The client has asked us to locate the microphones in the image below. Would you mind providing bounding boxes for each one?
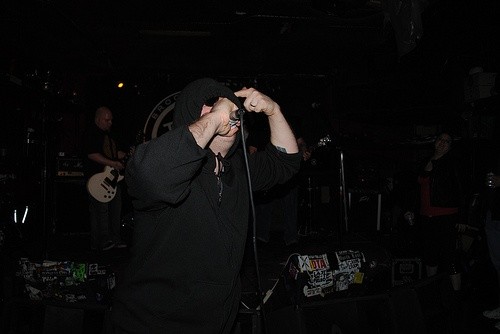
[230,109,245,121]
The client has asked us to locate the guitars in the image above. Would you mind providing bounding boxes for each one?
[301,134,330,159]
[87,136,150,203]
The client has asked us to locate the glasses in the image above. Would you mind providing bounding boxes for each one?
[205,96,219,106]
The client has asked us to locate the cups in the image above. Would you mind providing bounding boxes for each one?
[486,173,495,191]
[449,273,462,290]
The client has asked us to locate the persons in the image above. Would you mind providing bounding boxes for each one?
[111,78,304,334]
[254,79,311,251]
[83,106,135,250]
[398,134,500,329]
